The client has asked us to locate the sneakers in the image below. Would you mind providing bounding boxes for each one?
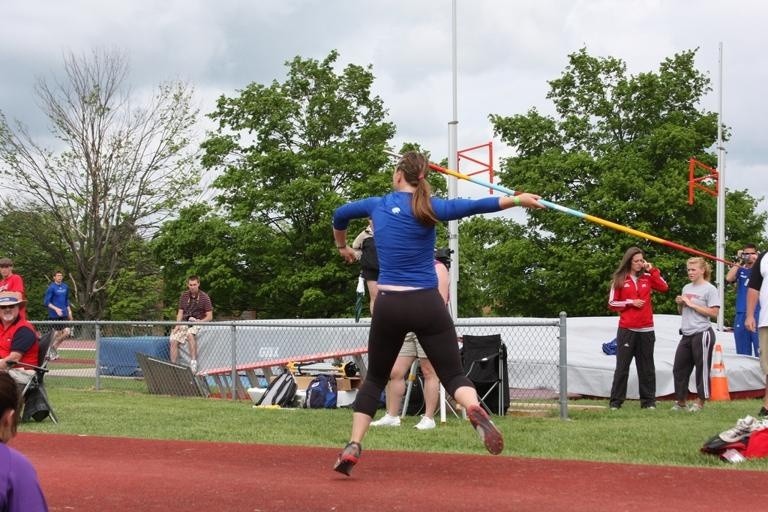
[190,360,198,374]
[688,403,704,412]
[465,404,505,456]
[758,407,768,418]
[719,415,761,443]
[413,417,436,430]
[47,348,60,361]
[369,412,401,427]
[332,441,361,476]
[670,403,688,412]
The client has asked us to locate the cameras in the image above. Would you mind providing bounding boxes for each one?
[69,324,74,337]
[742,253,749,260]
[186,315,195,327]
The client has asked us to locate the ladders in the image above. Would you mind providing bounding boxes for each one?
[194,347,368,401]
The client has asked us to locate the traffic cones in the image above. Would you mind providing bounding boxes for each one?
[708,344,733,401]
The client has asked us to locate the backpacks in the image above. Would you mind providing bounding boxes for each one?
[303,375,338,409]
[255,372,297,408]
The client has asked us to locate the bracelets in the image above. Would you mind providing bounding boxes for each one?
[513,193,521,208]
[335,242,348,251]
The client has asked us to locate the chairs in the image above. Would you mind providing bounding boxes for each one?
[0,326,61,427]
[385,331,510,421]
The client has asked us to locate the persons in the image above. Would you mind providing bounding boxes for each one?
[166,275,214,375]
[0,255,29,322]
[604,246,671,413]
[0,289,41,391]
[42,270,74,362]
[353,212,376,317]
[741,247,768,418]
[670,254,722,413]
[723,243,761,358]
[324,148,549,479]
[368,251,449,431]
[0,370,54,512]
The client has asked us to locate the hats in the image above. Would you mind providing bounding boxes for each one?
[0,291,28,307]
